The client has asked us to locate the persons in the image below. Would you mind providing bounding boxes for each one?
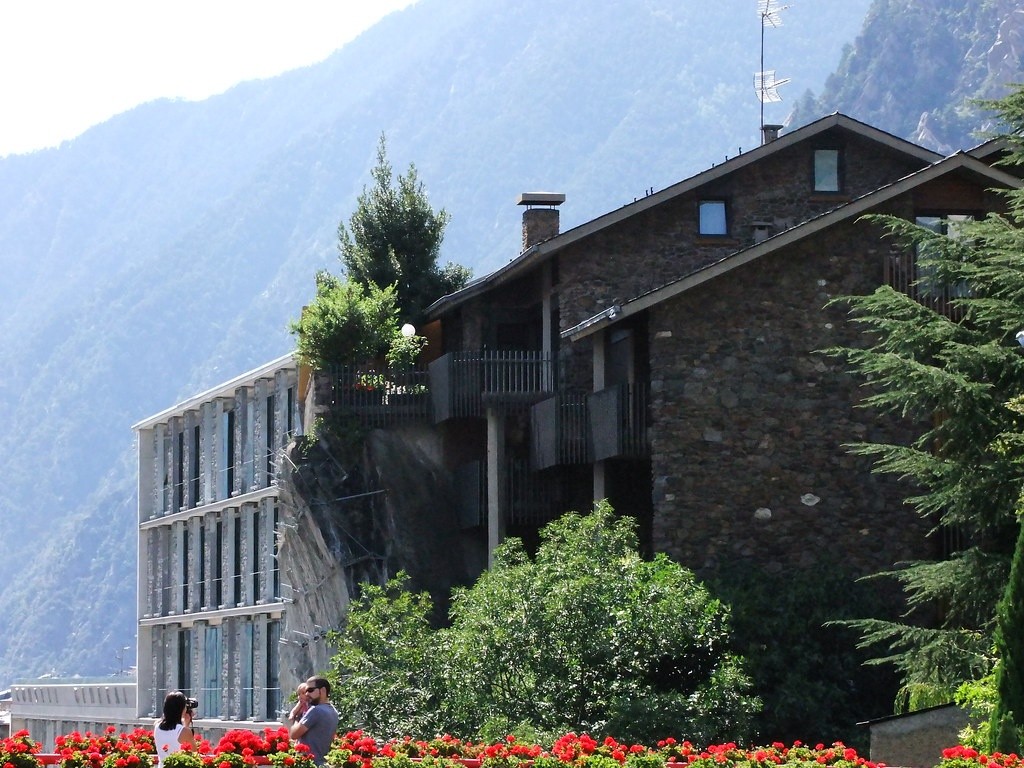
[288,676,339,768]
[154,691,197,768]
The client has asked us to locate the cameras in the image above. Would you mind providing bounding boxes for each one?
[186,697,199,714]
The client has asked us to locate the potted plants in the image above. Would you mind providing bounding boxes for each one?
[388,382,430,404]
[354,373,386,405]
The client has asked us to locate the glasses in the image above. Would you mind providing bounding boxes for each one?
[306,687,319,693]
